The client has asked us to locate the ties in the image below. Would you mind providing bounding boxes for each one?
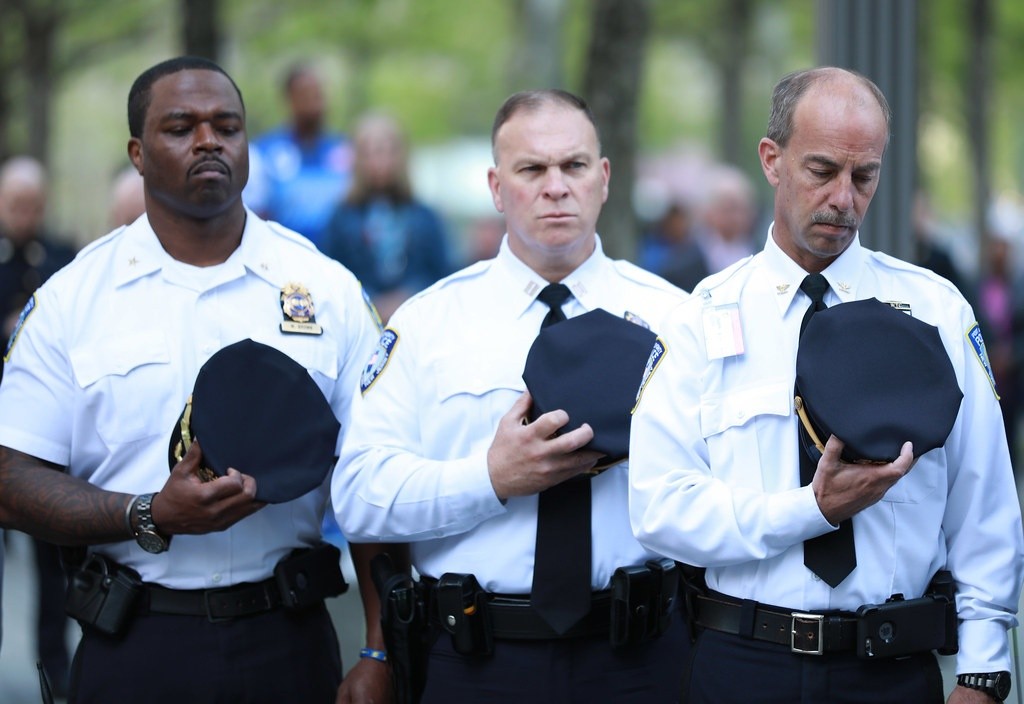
[531,283,595,634]
[799,273,857,589]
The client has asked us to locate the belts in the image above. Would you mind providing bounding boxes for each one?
[483,599,625,641]
[137,583,283,617]
[691,594,856,652]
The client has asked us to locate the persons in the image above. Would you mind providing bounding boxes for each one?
[247,64,506,324]
[629,67,1024,704]
[0,159,145,357]
[331,90,689,703]
[639,174,757,292]
[0,58,392,704]
[917,184,1024,458]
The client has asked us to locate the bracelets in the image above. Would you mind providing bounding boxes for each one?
[125,495,139,535]
[359,648,389,663]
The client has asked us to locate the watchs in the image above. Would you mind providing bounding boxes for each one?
[135,492,172,554]
[958,671,1011,701]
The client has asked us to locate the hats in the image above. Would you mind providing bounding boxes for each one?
[793,296,964,466]
[522,307,663,478]
[169,337,342,518]
